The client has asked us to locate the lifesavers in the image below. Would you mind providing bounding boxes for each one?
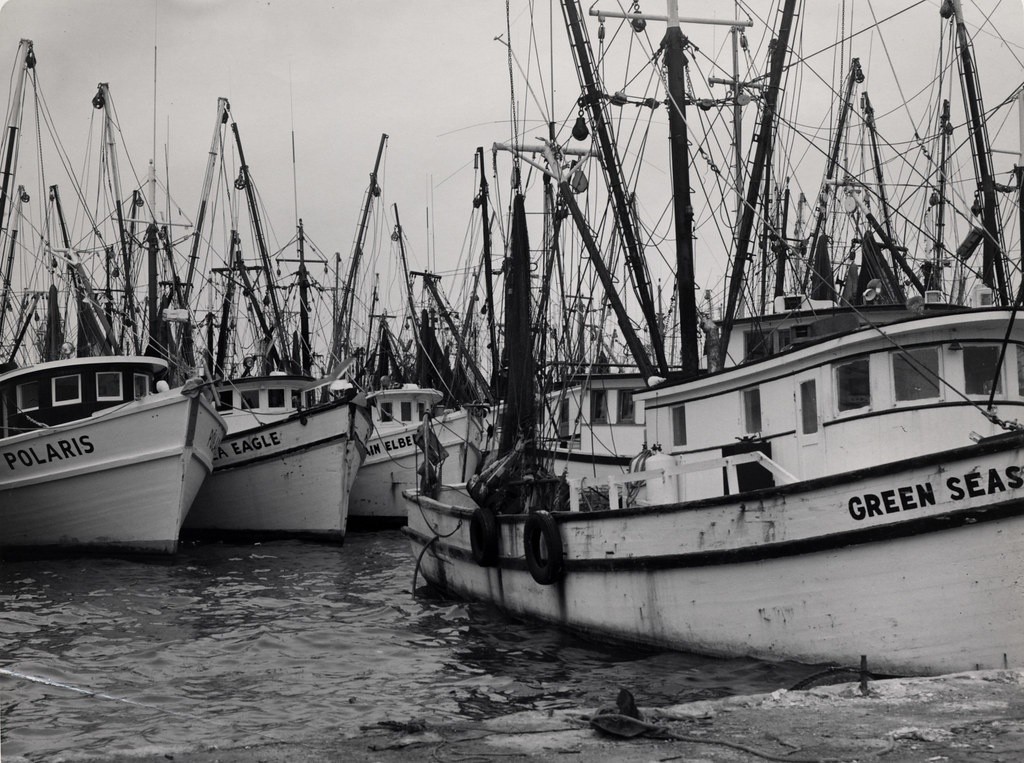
[523,508,564,585]
[468,506,500,568]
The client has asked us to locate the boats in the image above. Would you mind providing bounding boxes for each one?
[0,2,1023,676]
